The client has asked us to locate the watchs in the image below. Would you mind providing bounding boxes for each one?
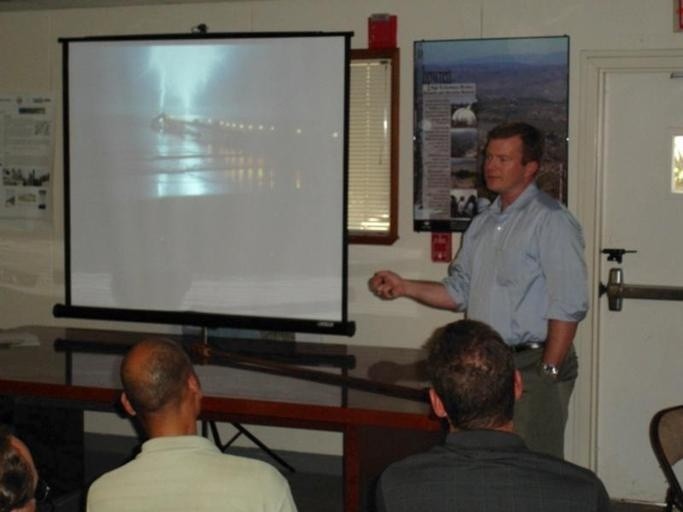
[538,359,561,377]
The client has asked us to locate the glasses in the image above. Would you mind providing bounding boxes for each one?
[29,480,50,504]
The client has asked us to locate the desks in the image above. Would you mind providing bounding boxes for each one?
[0,325,438,511]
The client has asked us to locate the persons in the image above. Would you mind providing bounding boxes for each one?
[366,122,590,461]
[373,319,612,511]
[0,428,44,512]
[84,339,298,512]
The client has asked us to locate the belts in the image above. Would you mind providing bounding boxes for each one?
[511,341,544,354]
